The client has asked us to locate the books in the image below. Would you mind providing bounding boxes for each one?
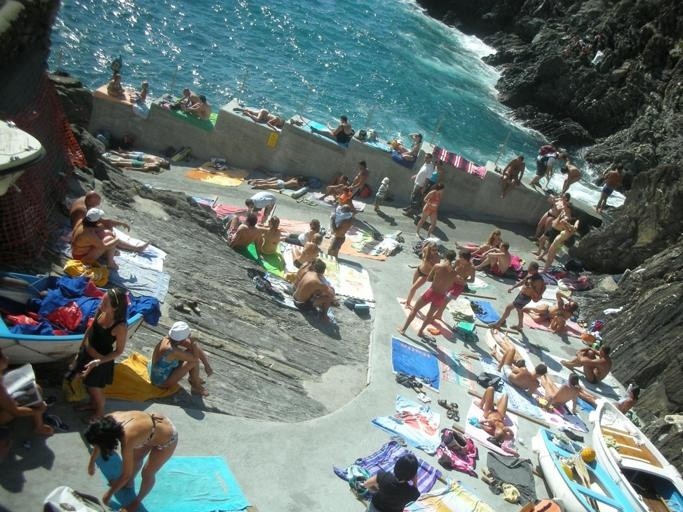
[2,362,43,408]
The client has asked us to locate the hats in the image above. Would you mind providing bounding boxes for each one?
[86,208,104,222]
[169,321,191,342]
[381,177,390,186]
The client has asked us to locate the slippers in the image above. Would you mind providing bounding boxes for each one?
[578,320,583,327]
[44,396,58,406]
[450,402,459,409]
[582,320,588,328]
[44,413,70,433]
[431,337,437,347]
[185,299,201,314]
[412,380,426,393]
[438,399,452,409]
[172,303,191,312]
[421,335,431,343]
[411,381,420,392]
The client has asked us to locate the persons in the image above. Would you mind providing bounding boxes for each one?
[491,344,549,398]
[593,161,624,212]
[149,321,213,395]
[77,288,128,422]
[454,229,511,276]
[375,177,390,211]
[292,258,338,323]
[501,156,526,196]
[490,327,526,368]
[401,153,442,218]
[245,192,276,225]
[577,381,640,414]
[103,72,211,172]
[399,239,440,309]
[281,218,320,246]
[432,251,475,320]
[396,250,459,337]
[292,233,323,268]
[364,454,421,512]
[562,24,606,59]
[249,176,305,190]
[257,216,281,256]
[474,386,514,442]
[309,118,352,144]
[71,190,150,253]
[561,345,613,383]
[541,372,582,414]
[327,205,352,257]
[319,176,349,207]
[83,410,178,512]
[70,208,123,270]
[344,161,368,214]
[485,260,543,330]
[417,183,444,237]
[0,348,55,436]
[12,387,35,406]
[231,105,285,132]
[393,132,422,162]
[530,149,581,195]
[224,213,262,261]
[523,291,578,330]
[529,193,581,272]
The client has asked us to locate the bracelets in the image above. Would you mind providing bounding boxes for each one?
[94,359,99,367]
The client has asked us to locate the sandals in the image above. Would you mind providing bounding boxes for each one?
[447,409,453,420]
[453,410,458,421]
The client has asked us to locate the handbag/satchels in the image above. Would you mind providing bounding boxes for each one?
[441,428,468,455]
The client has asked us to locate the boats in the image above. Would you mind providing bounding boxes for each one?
[534,428,632,512]
[0,271,159,364]
[591,396,682,512]
[0,117,48,197]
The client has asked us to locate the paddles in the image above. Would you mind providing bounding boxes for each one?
[537,397,570,421]
[572,456,598,512]
[578,456,600,512]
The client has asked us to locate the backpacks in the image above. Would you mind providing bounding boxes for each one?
[539,144,557,156]
